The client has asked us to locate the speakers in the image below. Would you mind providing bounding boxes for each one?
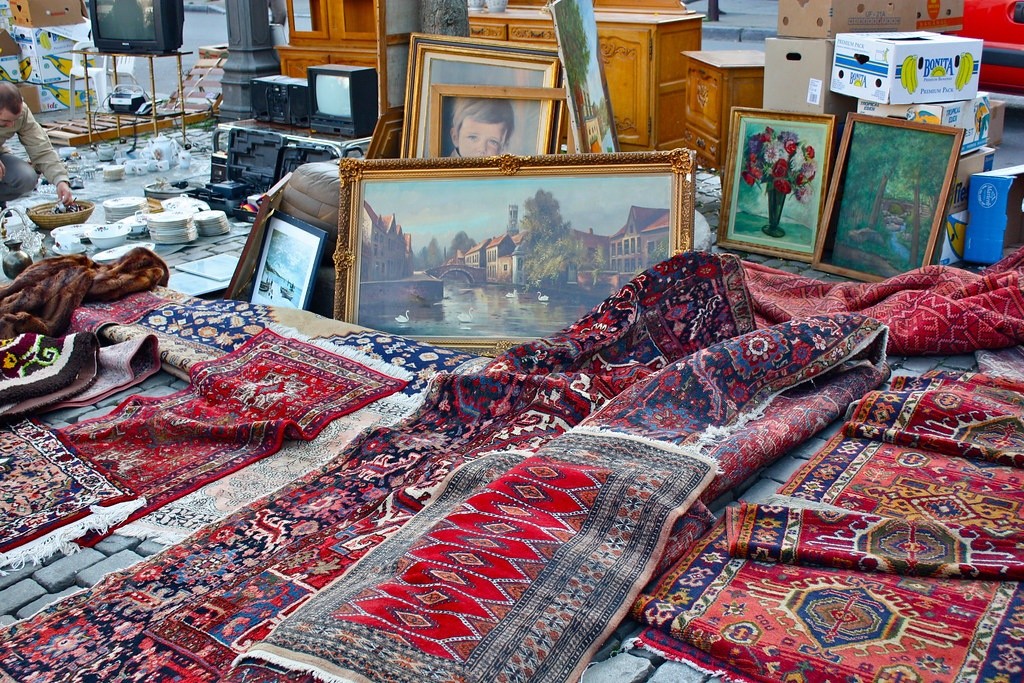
[250,75,290,121]
[286,80,311,128]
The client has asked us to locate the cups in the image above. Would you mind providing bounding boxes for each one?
[117,157,170,175]
[69,162,96,179]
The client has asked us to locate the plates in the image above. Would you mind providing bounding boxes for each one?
[52,244,87,256]
[93,242,156,264]
[103,180,231,244]
[50,224,97,241]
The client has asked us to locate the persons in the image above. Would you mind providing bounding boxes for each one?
[0,81,74,217]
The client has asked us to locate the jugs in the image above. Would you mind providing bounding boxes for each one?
[147,133,178,164]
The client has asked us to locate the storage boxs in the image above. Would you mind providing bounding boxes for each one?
[761,0,1024,265]
[198,43,229,60]
[0,0,95,113]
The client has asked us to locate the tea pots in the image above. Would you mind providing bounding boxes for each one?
[90,144,117,162]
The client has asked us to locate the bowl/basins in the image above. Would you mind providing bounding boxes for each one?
[89,225,131,249]
[26,201,95,229]
[57,236,82,250]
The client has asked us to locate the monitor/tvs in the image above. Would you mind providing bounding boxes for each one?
[90,0,184,54]
[306,64,379,139]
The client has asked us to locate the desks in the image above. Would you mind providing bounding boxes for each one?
[69,50,194,146]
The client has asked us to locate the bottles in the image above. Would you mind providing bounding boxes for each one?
[2,240,33,280]
[179,148,191,169]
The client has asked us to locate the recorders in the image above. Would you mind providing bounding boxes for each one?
[108,84,153,115]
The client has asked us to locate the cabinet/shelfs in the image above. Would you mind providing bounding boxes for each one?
[467,0,706,152]
[680,50,764,170]
[273,0,378,119]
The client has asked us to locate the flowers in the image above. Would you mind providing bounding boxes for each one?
[740,124,817,203]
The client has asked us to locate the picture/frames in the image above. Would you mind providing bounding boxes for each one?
[717,103,836,263]
[223,172,330,310]
[335,32,697,356]
[813,112,966,284]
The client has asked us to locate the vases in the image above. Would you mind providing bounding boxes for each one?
[761,181,787,238]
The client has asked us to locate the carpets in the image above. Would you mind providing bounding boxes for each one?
[1,251,1024,683]
